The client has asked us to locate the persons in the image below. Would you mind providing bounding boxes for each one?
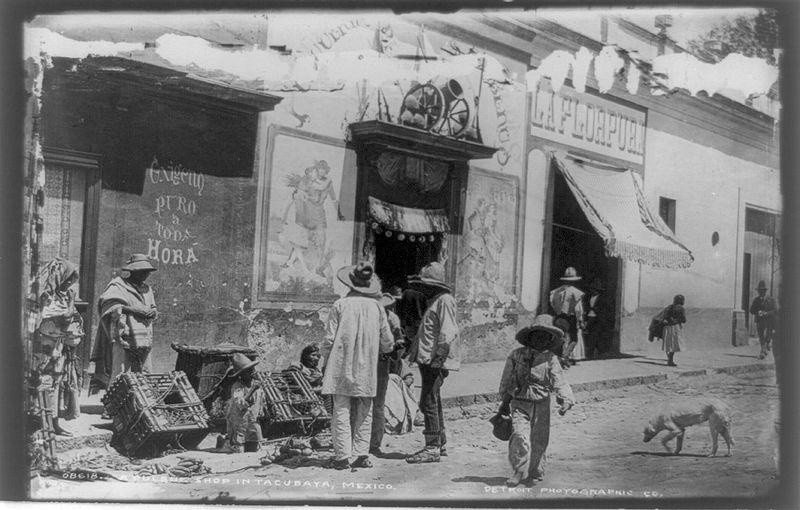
[552,266,618,362]
[497,323,575,486]
[278,160,338,273]
[25,254,457,471]
[749,277,783,362]
[657,293,688,369]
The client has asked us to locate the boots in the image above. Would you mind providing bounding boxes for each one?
[406,430,441,463]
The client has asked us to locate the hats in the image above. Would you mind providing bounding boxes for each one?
[755,280,767,290]
[120,253,158,274]
[560,267,582,282]
[338,262,381,295]
[515,314,564,348]
[406,261,453,294]
[229,350,259,378]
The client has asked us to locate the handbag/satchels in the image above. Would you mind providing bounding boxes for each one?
[489,415,513,441]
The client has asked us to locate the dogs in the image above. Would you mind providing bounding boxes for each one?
[640,397,737,458]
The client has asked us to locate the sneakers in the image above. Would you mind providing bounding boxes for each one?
[507,471,523,486]
